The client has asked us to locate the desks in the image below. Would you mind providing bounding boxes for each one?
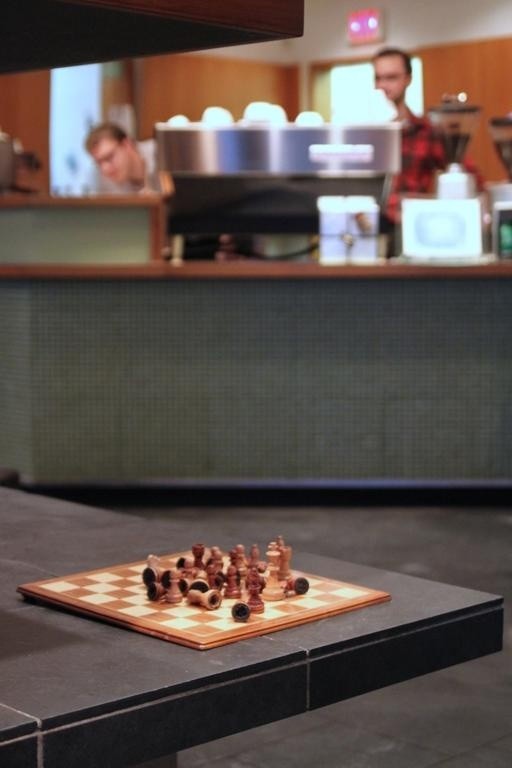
[1,484,503,768]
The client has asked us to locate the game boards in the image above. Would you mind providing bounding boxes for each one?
[16,545,393,654]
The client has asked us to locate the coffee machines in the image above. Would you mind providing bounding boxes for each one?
[425,91,511,204]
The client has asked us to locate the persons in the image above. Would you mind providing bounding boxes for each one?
[372,48,485,258]
[79,123,158,194]
